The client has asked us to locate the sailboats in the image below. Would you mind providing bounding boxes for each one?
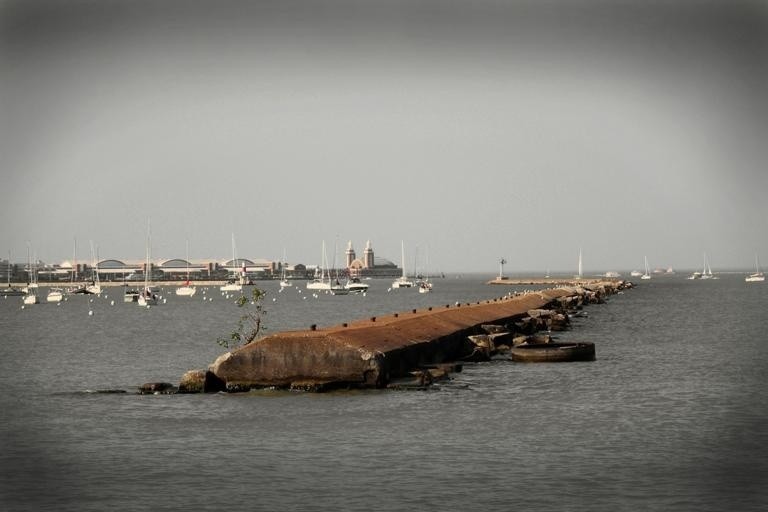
[306,241,331,290]
[327,246,349,296]
[175,239,196,297]
[65,239,104,295]
[21,246,39,306]
[3,243,26,297]
[122,232,162,307]
[279,249,294,288]
[219,234,243,292]
[495,249,765,286]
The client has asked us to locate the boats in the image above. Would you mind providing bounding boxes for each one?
[224,274,235,283]
[46,291,64,303]
[345,276,370,294]
[391,240,416,290]
[414,273,433,294]
[239,276,254,286]
